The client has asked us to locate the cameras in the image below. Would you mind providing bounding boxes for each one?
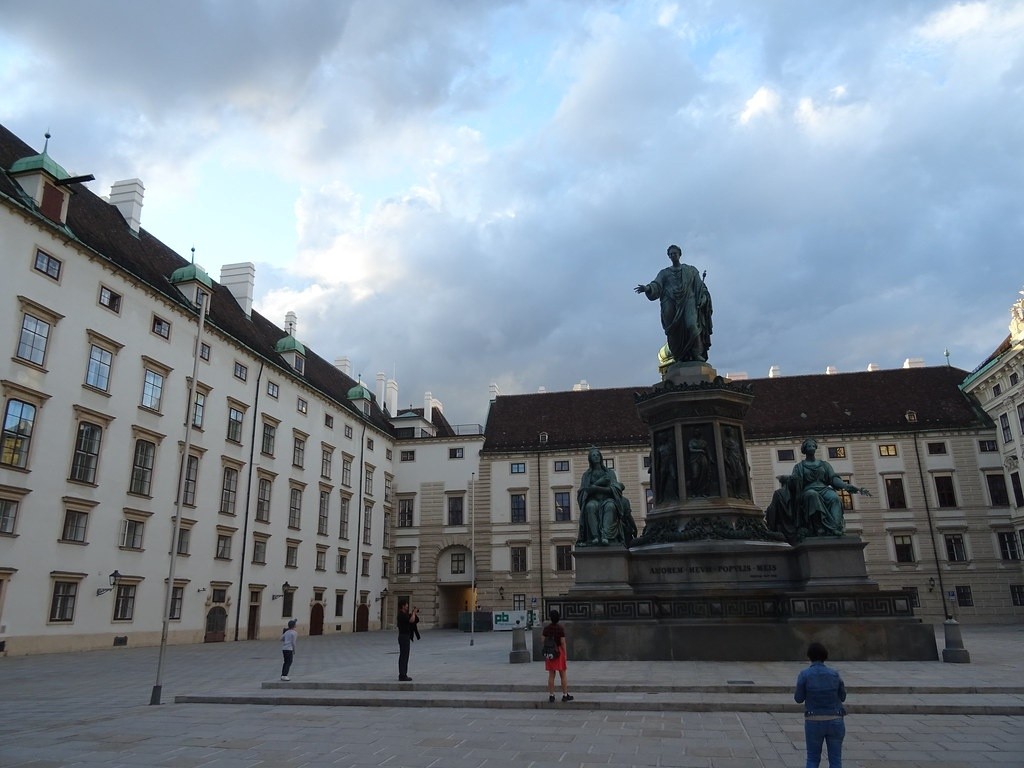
[414,607,419,612]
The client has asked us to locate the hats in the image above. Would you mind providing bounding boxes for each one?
[288,619,297,628]
[549,610,560,623]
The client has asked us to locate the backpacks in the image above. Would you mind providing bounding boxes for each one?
[542,624,560,659]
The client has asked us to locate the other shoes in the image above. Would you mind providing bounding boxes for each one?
[280,675,290,680]
[399,674,411,680]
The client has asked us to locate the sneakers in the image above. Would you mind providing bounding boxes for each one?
[549,695,555,701]
[562,693,574,702]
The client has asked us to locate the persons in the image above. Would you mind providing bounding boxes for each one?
[577,448,626,547]
[280,619,298,681]
[541,609,574,703]
[634,244,709,363]
[775,436,858,537]
[397,599,420,682]
[794,641,846,768]
